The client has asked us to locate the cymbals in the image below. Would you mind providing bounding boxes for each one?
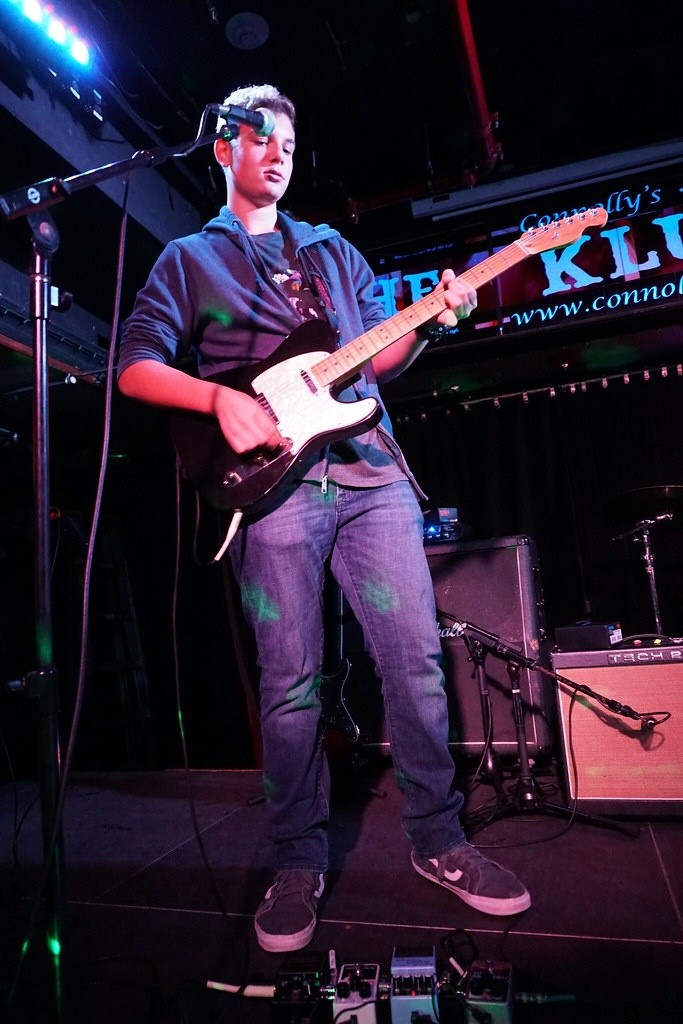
[598,483,683,532]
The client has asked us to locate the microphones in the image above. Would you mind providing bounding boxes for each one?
[209,103,276,138]
[641,715,657,731]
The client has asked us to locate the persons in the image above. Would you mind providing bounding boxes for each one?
[116,85,531,954]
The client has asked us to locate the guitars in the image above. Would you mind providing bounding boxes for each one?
[166,201,613,524]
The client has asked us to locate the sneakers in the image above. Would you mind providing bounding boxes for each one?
[410,840,533,917]
[254,863,326,951]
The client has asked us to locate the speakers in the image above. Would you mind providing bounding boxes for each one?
[548,644,683,818]
[334,532,557,764]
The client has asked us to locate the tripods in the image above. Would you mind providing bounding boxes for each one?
[431,609,643,842]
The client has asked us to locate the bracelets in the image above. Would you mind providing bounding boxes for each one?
[209,384,223,414]
